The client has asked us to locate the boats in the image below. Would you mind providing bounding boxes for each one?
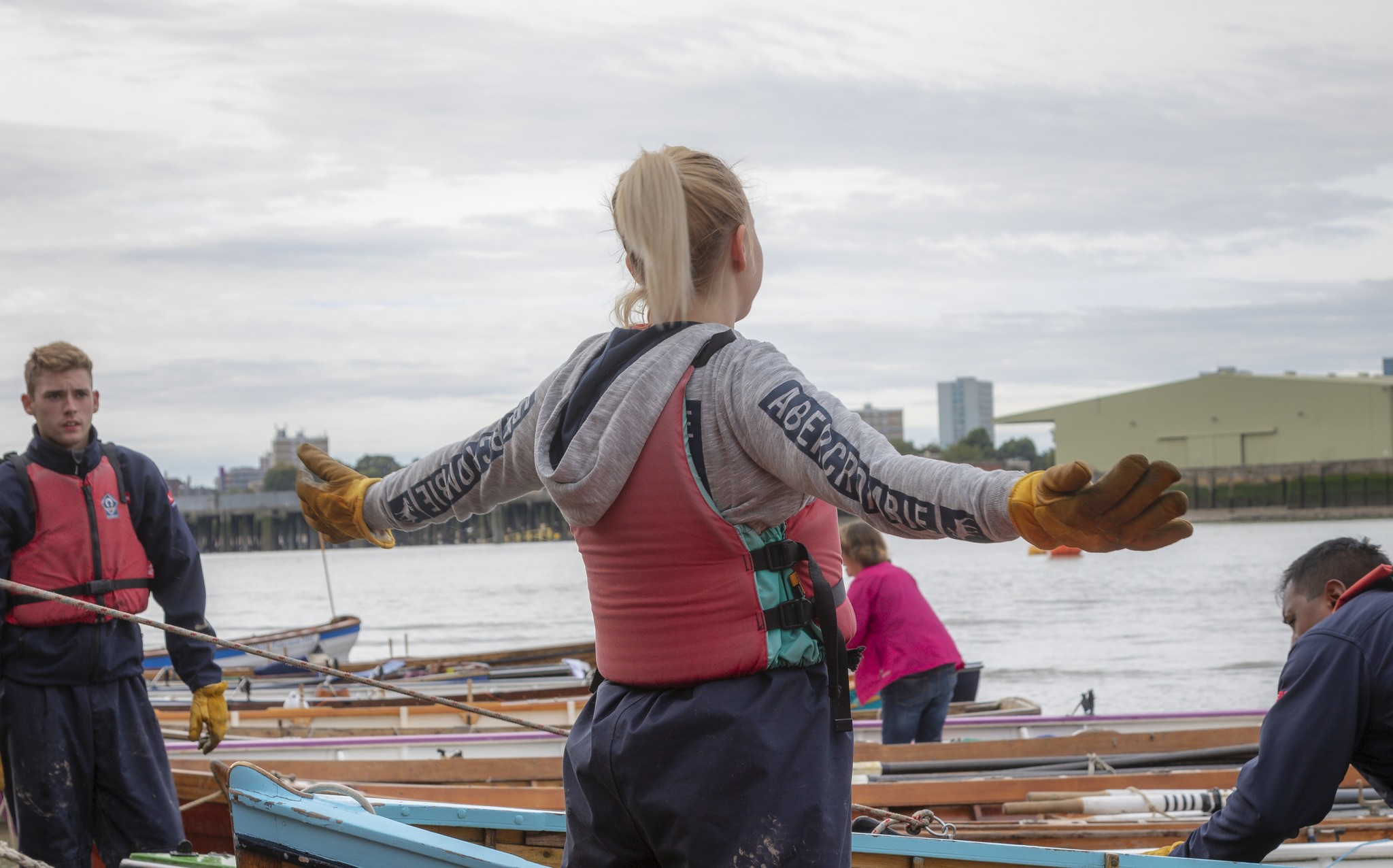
[137,608,1393,866]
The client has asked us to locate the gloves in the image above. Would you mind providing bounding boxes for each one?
[1140,839,1185,857]
[296,443,396,550]
[188,681,230,756]
[1006,453,1193,553]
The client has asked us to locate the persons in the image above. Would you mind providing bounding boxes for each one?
[0,339,229,868]
[835,520,966,746]
[297,148,1195,868]
[1138,535,1393,863]
